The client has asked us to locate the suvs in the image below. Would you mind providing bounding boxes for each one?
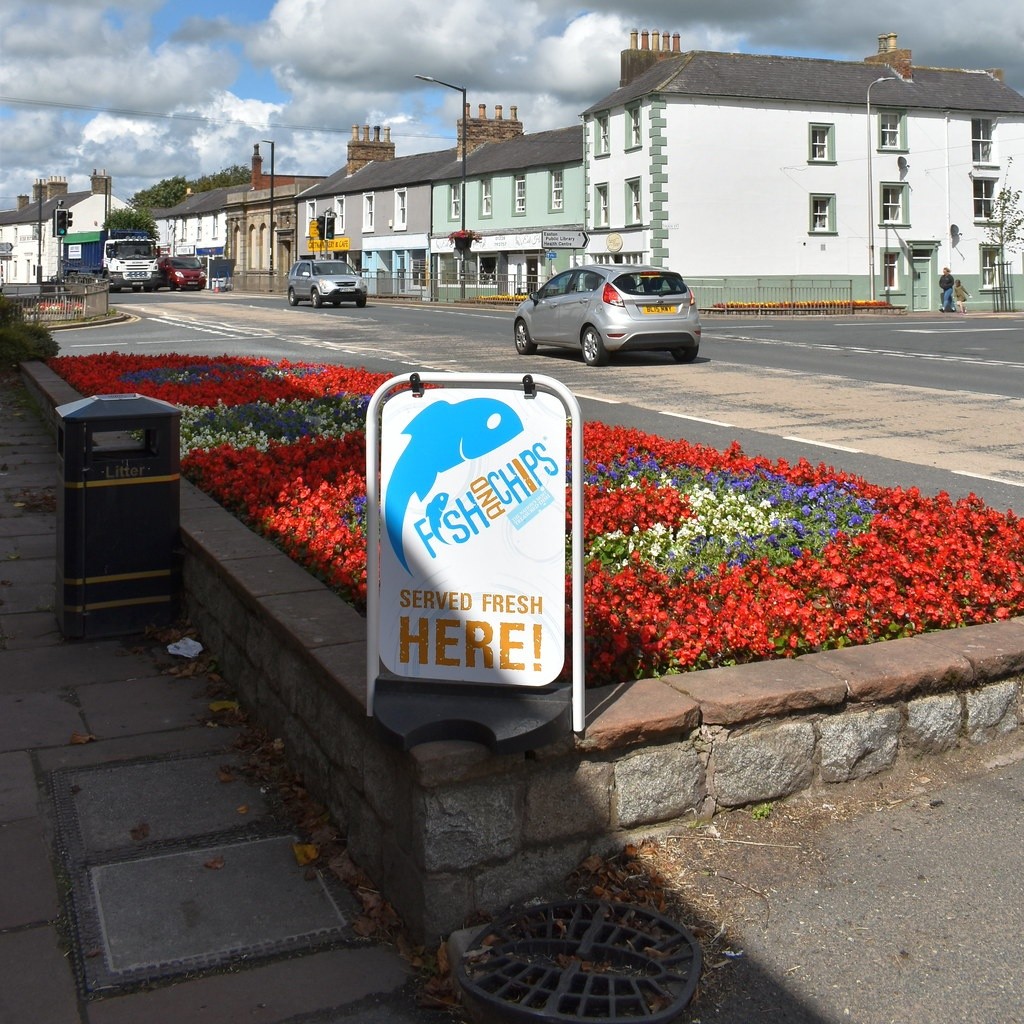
[286,260,368,309]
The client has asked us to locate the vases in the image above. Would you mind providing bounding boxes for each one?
[452,237,475,251]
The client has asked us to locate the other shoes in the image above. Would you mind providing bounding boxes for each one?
[939,307,968,314]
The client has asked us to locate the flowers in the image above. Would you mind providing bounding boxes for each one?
[447,230,482,246]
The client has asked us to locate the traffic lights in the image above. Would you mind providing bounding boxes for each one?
[316,216,325,240]
[57,210,66,236]
[327,217,335,239]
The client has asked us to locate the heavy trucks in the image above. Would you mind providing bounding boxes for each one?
[62,229,159,293]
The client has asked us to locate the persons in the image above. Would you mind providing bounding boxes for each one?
[953,280,971,316]
[938,267,956,313]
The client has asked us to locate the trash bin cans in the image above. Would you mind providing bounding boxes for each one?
[54,392,186,644]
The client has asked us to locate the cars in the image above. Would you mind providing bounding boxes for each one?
[158,255,207,291]
[512,264,702,367]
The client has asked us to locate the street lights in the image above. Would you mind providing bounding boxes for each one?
[262,138,275,276]
[413,73,468,301]
[865,76,898,300]
[96,176,109,228]
